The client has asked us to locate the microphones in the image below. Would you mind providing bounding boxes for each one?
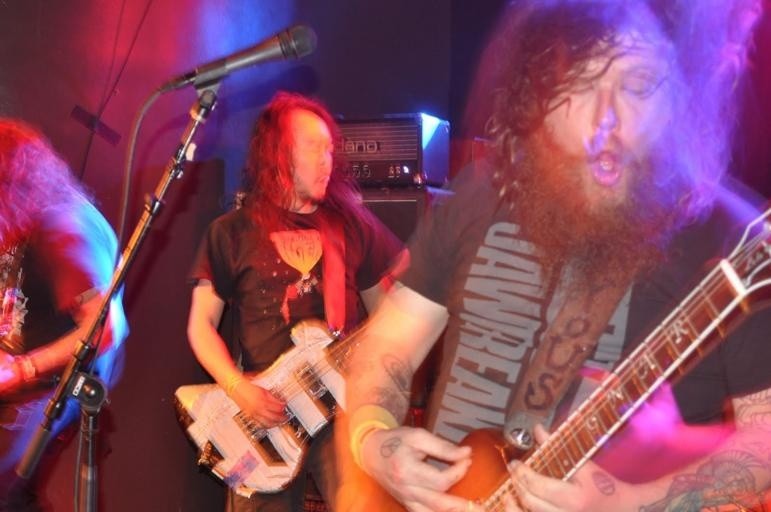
[158,24,319,94]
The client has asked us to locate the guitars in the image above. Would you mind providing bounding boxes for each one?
[335,207,771,512]
[170,299,385,499]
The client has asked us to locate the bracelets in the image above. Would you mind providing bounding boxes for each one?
[348,399,398,472]
[17,352,38,386]
[227,374,250,396]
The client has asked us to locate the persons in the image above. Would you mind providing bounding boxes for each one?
[1,119,130,511]
[338,0,771,512]
[184,90,413,512]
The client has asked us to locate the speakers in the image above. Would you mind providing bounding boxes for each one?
[229,186,458,512]
